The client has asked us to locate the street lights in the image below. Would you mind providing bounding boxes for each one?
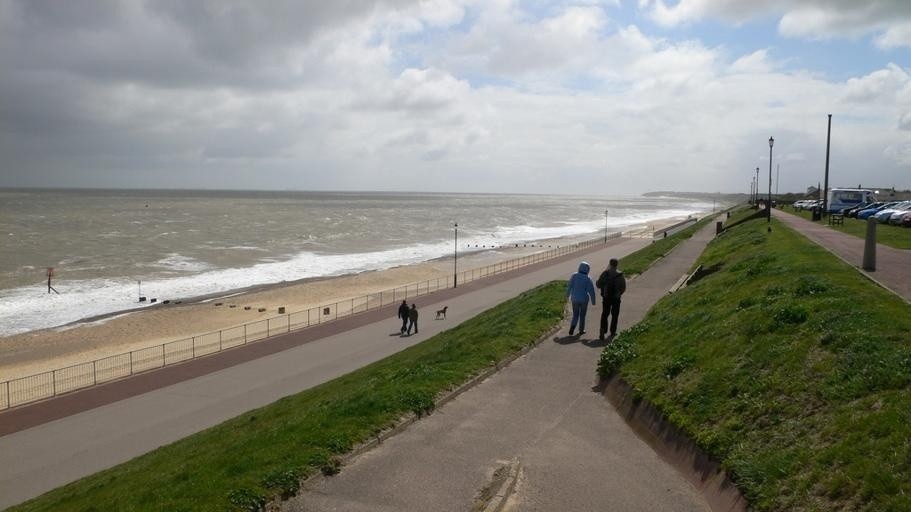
[766,136,776,222]
[751,168,761,208]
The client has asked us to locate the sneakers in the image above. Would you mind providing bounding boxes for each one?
[400,328,418,335]
[599,332,618,340]
[568,329,587,335]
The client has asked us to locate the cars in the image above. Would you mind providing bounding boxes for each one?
[840,200,911,227]
[793,198,824,211]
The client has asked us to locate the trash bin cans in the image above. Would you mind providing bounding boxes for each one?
[717,222,723,234]
[728,212,730,218]
[813,207,822,220]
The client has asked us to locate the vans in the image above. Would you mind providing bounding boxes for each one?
[827,187,879,214]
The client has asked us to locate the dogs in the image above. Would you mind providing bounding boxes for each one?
[435,306,448,319]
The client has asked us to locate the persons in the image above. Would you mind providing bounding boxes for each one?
[566,258,626,339]
[398,300,418,336]
